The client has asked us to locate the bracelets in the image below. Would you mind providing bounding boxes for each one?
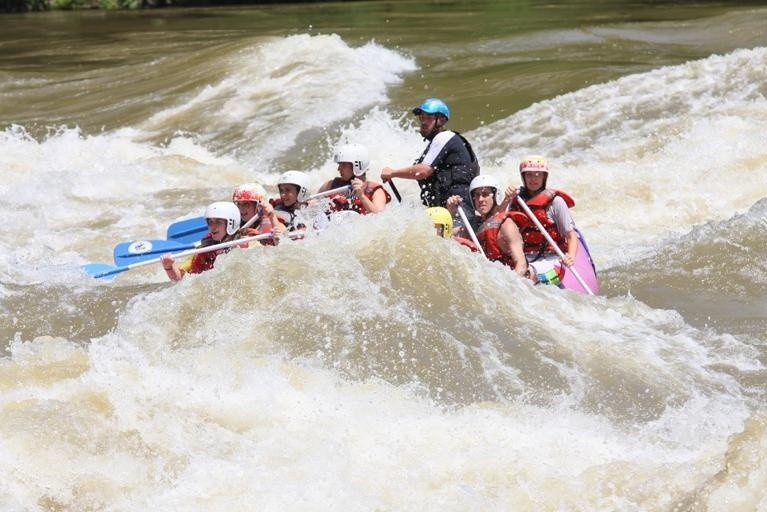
[504,199,509,203]
[165,268,172,271]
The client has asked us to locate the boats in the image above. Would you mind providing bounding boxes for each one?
[519,222,602,302]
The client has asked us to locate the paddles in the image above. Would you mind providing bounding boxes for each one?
[79,184,353,280]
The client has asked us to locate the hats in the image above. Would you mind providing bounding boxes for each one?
[468,174,506,210]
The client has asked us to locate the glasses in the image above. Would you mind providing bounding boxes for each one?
[525,173,544,178]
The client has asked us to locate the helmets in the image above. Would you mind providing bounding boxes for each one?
[519,155,549,188]
[425,206,453,239]
[203,201,241,235]
[232,183,268,215]
[277,170,313,204]
[412,97,449,119]
[333,143,371,176]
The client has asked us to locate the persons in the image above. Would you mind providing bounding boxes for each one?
[160,200,284,283]
[424,206,479,255]
[497,155,578,286]
[273,170,330,241]
[380,98,481,238]
[316,142,392,222]
[232,182,287,246]
[445,173,541,288]
